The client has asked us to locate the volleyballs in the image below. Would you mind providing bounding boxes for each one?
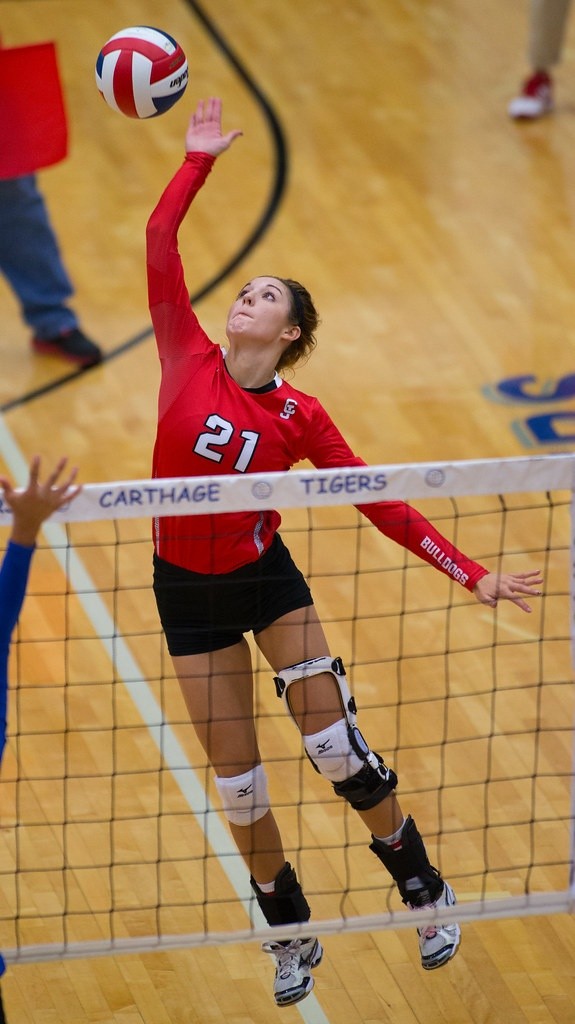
[95,24,190,121]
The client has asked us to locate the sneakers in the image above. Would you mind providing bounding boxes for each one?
[402,881,462,970]
[507,72,554,120]
[261,923,323,1007]
[32,328,103,367]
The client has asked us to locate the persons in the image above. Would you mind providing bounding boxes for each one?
[2,42,106,368]
[506,1,569,121]
[4,454,85,751]
[142,94,545,1008]
